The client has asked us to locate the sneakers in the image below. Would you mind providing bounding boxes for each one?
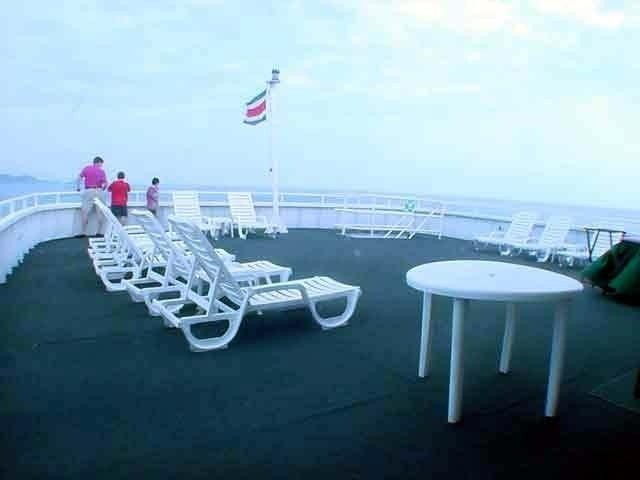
[74,234,85,238]
[96,233,104,237]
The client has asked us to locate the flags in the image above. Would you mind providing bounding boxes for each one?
[242,88,266,124]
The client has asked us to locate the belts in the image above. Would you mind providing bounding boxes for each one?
[85,185,102,188]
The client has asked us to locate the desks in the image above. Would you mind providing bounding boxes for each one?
[205,216,234,238]
[404,257,585,426]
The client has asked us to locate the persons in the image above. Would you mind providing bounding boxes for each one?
[76,156,110,237]
[146,177,160,216]
[107,171,131,216]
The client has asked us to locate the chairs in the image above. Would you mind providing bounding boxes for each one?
[172,191,217,241]
[227,191,277,239]
[335,197,627,268]
[120,209,294,317]
[98,206,236,296]
[152,218,363,352]
[88,198,187,276]
